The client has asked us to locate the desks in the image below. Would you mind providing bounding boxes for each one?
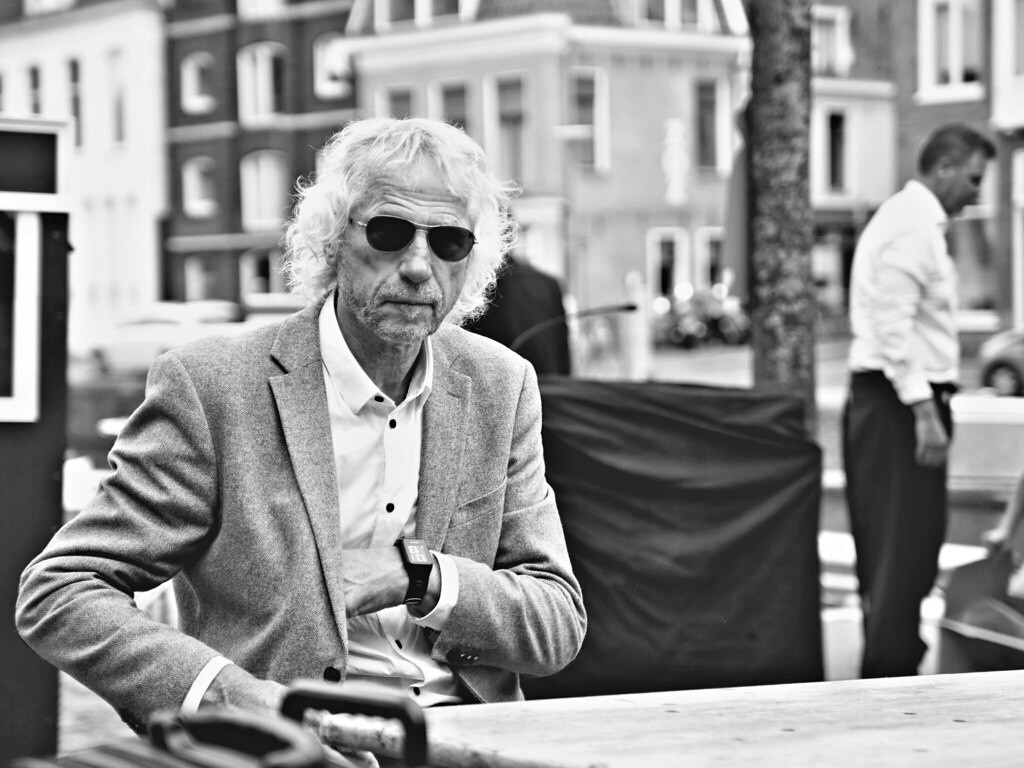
[316,669,1024,768]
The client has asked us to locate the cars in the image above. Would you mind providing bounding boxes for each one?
[974,327,1023,396]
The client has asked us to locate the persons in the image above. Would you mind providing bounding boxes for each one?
[461,250,572,377]
[13,116,587,768]
[841,123,997,679]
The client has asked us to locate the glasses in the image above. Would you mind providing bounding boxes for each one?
[347,215,480,262]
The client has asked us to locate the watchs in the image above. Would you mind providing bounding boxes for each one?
[393,536,433,605]
